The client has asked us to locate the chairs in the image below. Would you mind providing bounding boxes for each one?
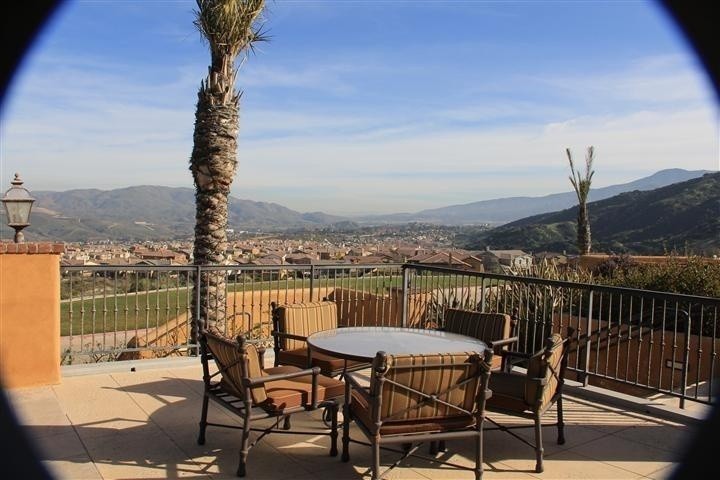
[439,327,575,474]
[435,309,510,371]
[196,319,346,478]
[340,347,493,480]
[271,297,371,378]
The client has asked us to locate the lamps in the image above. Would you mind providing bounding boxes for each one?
[1,173,35,243]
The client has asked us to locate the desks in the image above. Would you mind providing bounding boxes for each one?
[307,325,488,463]
